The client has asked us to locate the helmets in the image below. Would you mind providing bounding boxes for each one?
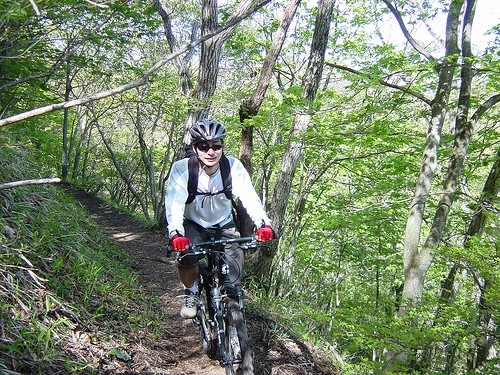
[188,118,227,145]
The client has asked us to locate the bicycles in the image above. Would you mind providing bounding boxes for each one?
[166,232,281,375]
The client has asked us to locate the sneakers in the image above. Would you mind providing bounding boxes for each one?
[175,289,200,318]
[230,336,241,359]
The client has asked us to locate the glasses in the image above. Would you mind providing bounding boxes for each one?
[196,144,223,151]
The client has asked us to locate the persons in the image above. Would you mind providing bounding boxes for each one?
[165,119,276,359]
